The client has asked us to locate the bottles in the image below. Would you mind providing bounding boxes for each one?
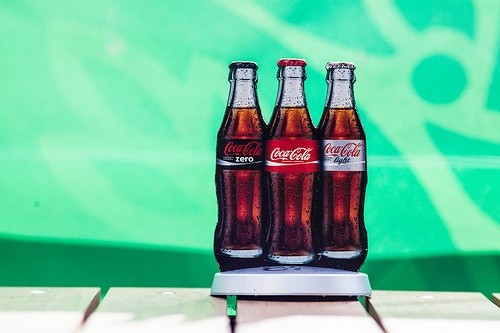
[264,58,319,265]
[312,60,369,271]
[213,61,268,273]
